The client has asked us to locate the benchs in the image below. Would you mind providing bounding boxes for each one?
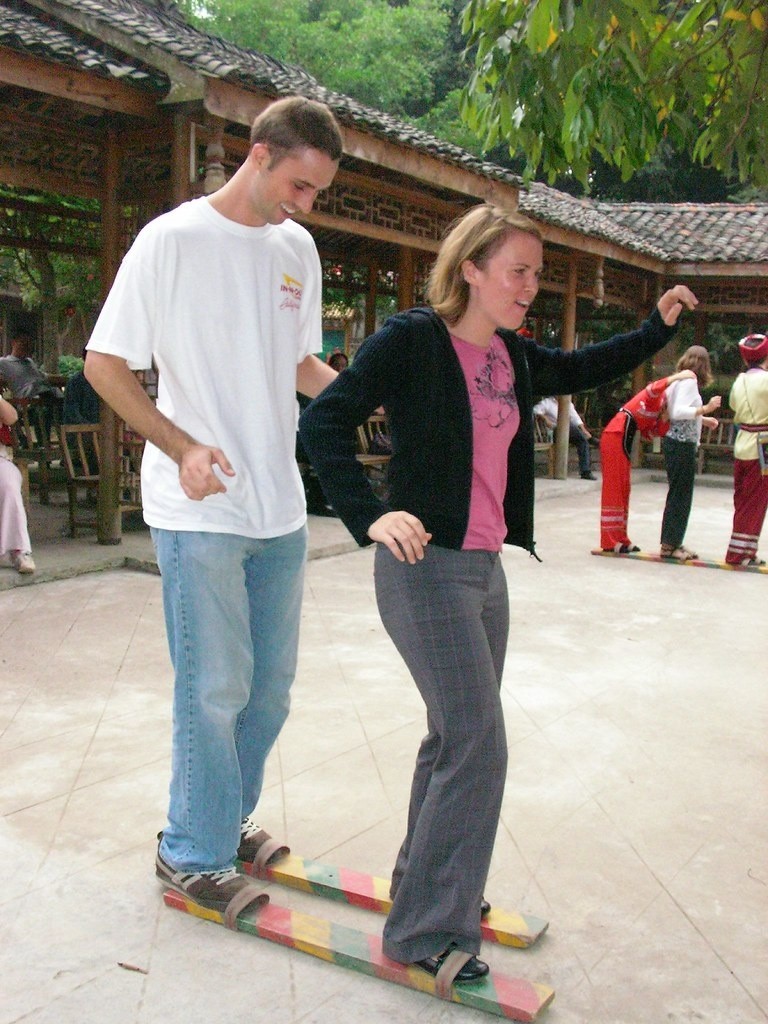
[356,415,392,465]
[57,423,145,537]
[7,397,81,505]
[696,418,737,475]
[534,414,555,478]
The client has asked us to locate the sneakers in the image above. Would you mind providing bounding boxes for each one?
[154,816,284,915]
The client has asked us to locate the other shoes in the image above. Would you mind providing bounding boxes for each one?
[741,556,766,565]
[603,544,640,553]
[588,437,600,445]
[10,548,37,574]
[581,470,597,480]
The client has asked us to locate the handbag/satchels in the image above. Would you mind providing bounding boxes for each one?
[758,435,768,477]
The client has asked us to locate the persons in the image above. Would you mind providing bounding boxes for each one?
[533,394,600,480]
[0,329,71,574]
[599,370,698,555]
[657,345,722,561]
[725,334,768,568]
[59,339,119,506]
[328,352,349,372]
[83,95,386,920]
[297,206,698,986]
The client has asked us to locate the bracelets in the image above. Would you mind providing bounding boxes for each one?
[701,405,705,413]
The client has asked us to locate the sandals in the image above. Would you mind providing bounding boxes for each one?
[660,544,698,561]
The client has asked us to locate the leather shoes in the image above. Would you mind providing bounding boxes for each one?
[480,896,491,917]
[414,944,491,985]
